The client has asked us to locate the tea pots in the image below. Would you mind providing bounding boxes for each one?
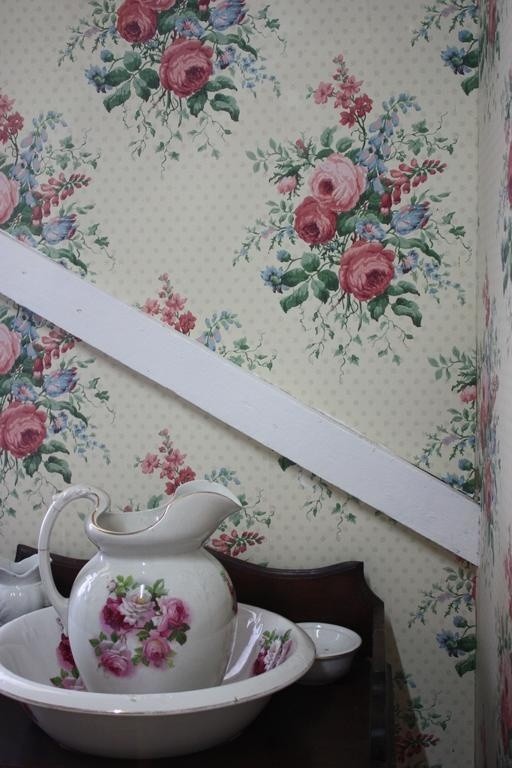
[37,475,243,694]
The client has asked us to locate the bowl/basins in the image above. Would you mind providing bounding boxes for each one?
[299,622,361,684]
[0,601,316,757]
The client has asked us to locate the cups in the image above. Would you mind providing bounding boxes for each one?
[1,549,52,618]
